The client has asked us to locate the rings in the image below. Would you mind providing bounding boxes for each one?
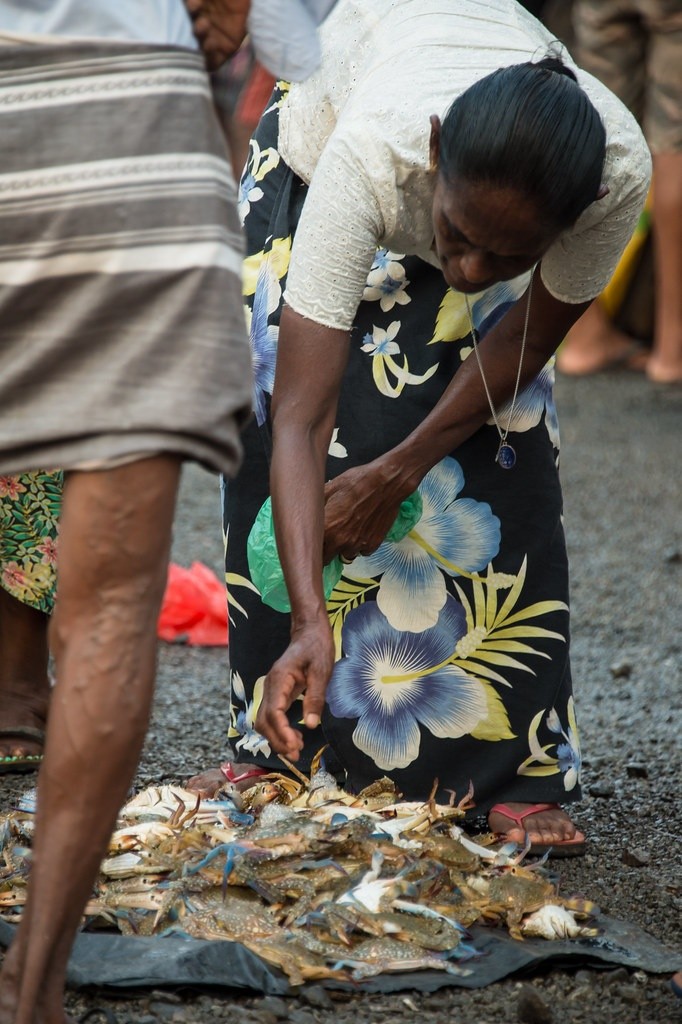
[339,553,353,565]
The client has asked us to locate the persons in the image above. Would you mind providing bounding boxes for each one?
[210,1,682,384]
[179,2,654,855]
[1,0,256,1024]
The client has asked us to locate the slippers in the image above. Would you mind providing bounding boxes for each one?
[487,801,587,856]
[0,724,45,773]
[186,761,270,800]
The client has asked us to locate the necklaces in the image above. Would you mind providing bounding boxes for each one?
[464,264,533,469]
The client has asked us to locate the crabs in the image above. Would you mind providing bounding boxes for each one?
[1,746,601,981]
